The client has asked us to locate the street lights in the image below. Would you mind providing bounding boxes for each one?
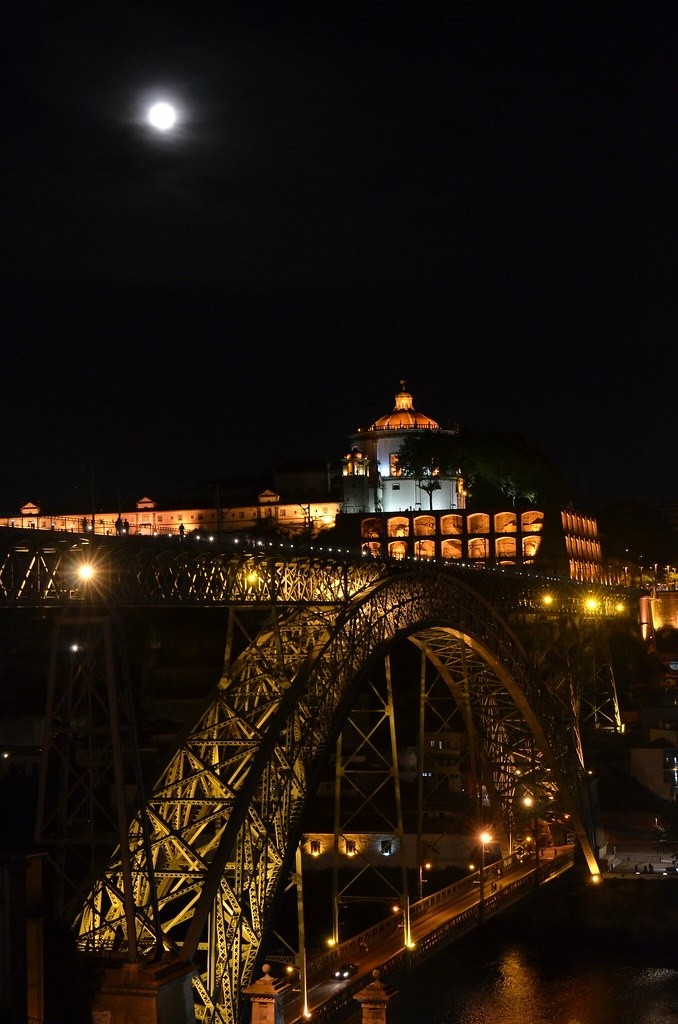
[524,794,543,869]
[479,830,494,902]
[392,906,409,947]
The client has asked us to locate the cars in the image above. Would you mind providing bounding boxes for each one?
[333,962,360,980]
